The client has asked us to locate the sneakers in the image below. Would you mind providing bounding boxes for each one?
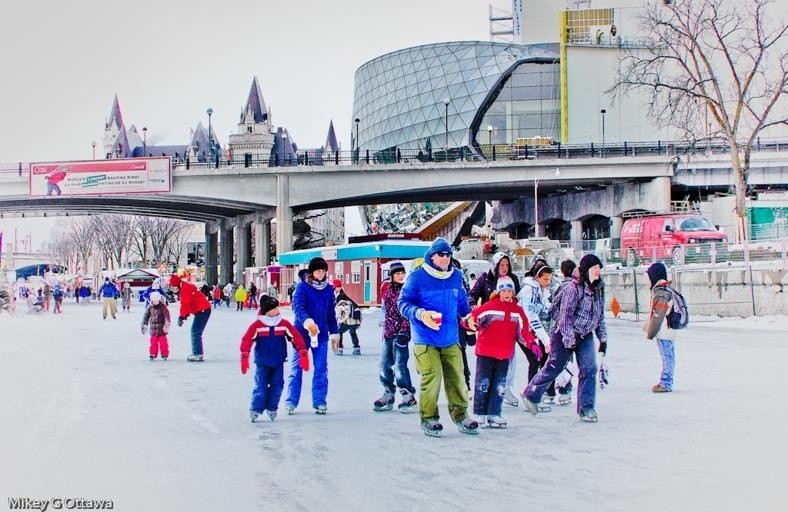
[373,382,669,431]
[150,354,203,361]
[249,403,327,419]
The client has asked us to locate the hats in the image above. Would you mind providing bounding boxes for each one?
[150,292,161,300]
[308,258,328,273]
[579,254,600,275]
[389,262,405,276]
[333,280,342,286]
[260,295,279,314]
[429,237,453,257]
[170,275,181,287]
[496,276,515,295]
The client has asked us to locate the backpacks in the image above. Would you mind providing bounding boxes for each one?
[653,285,688,329]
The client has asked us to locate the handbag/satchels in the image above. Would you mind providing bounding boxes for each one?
[349,303,361,319]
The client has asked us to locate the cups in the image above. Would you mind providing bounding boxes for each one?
[433,314,441,326]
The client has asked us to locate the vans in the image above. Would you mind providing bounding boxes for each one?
[378,258,492,293]
[456,234,620,285]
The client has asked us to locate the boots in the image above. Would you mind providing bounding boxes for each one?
[352,348,360,355]
[335,348,343,355]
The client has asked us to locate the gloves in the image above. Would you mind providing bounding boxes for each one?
[421,310,441,330]
[297,349,309,371]
[531,344,543,362]
[303,317,318,337]
[598,343,606,356]
[241,351,250,374]
[141,315,188,335]
[331,333,340,352]
[394,334,408,351]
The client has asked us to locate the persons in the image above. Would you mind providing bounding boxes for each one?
[44,166,70,196]
[643,263,675,394]
[23,256,364,422]
[373,234,610,437]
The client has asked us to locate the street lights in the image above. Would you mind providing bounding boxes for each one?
[207,108,214,150]
[282,134,287,161]
[487,125,494,160]
[600,108,607,157]
[143,127,149,156]
[534,168,561,238]
[92,140,96,160]
[444,97,451,163]
[355,118,360,165]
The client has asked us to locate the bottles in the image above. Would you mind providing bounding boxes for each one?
[310,335,319,348]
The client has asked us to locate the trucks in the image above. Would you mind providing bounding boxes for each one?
[619,214,730,265]
[511,137,568,159]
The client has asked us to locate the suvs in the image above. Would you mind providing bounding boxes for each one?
[508,144,536,160]
